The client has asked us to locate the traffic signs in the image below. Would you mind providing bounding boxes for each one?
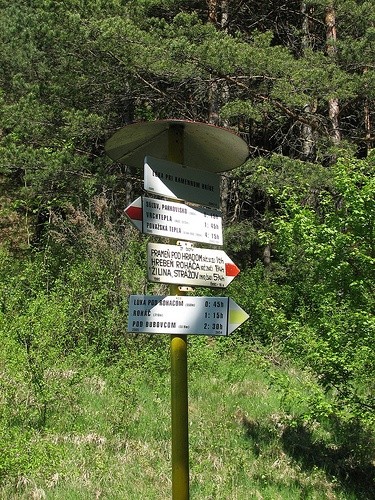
[124,156,250,335]
